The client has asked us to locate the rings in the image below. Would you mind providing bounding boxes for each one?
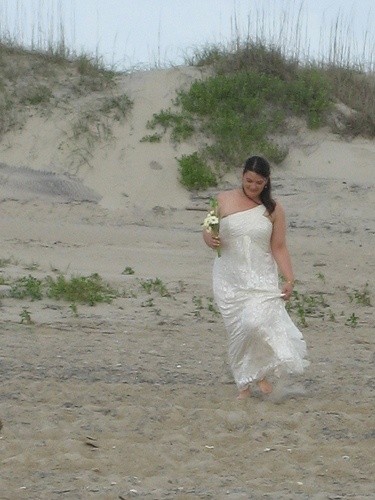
[215,236,218,239]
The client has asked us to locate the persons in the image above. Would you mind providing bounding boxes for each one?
[202,156,311,400]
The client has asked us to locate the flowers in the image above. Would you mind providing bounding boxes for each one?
[200,194,221,257]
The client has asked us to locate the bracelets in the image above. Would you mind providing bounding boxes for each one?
[286,280,295,289]
